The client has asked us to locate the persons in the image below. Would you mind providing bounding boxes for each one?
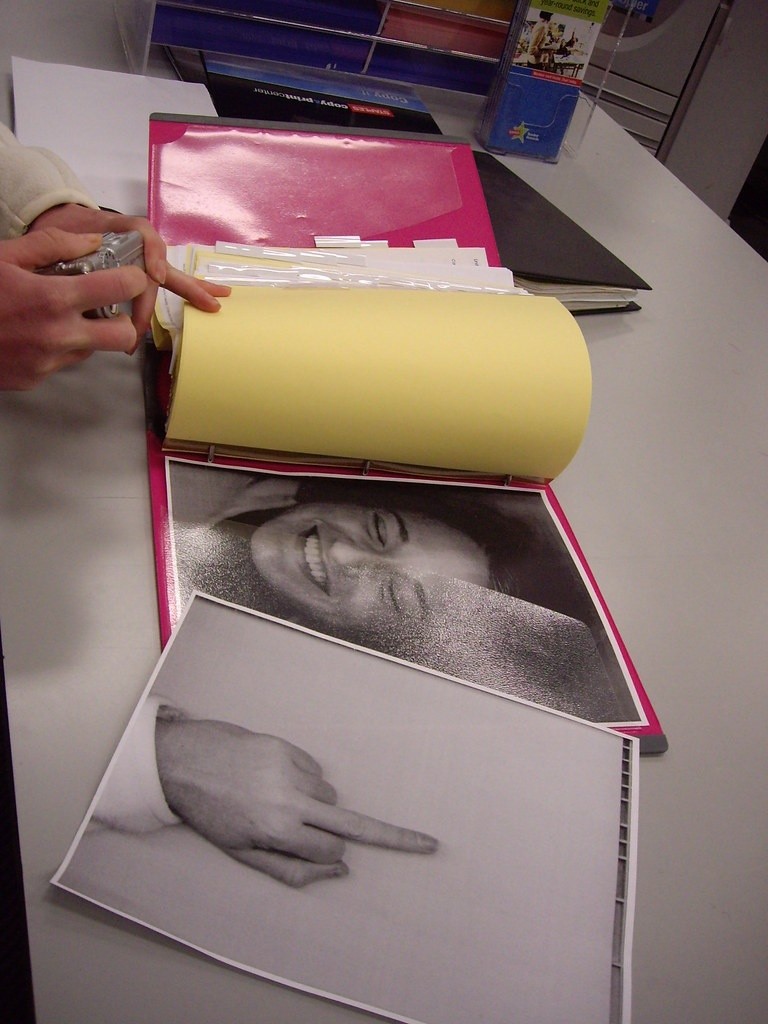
[0,120,231,392]
[527,10,555,69]
[85,695,439,889]
[170,461,515,633]
[557,27,579,55]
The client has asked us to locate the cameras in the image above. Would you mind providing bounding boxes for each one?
[34,231,145,320]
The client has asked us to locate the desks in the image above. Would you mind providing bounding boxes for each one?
[0,0,768,1024]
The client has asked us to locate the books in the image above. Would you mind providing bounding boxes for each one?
[138,112,670,756]
[478,0,613,160]
[474,150,654,316]
[165,46,444,135]
